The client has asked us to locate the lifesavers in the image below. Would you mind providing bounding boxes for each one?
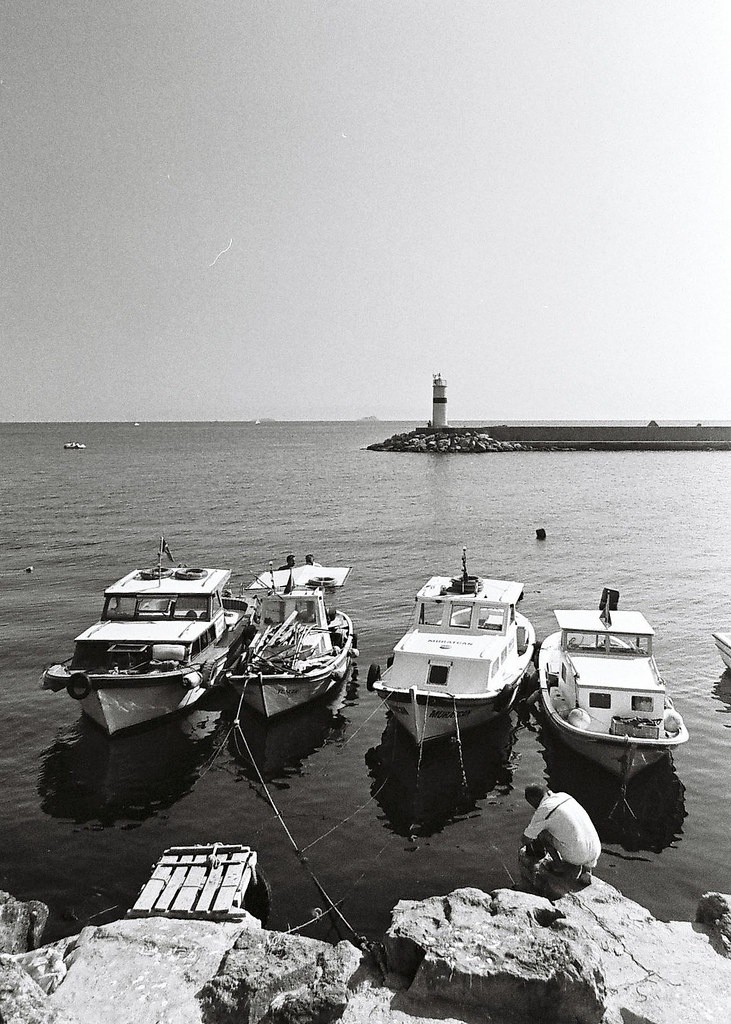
[201,659,218,687]
[308,576,337,587]
[452,575,484,594]
[67,673,94,700]
[499,683,514,706]
[175,568,208,580]
[141,567,174,580]
[367,663,380,692]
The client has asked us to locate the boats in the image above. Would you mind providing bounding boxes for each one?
[41,569,251,739]
[368,549,537,746]
[63,442,87,448]
[711,632,731,671]
[222,566,358,716]
[540,584,690,779]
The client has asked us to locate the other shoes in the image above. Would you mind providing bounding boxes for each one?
[577,866,592,887]
[550,862,566,875]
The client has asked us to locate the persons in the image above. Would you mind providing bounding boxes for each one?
[277,555,295,570]
[524,784,601,884]
[305,554,322,567]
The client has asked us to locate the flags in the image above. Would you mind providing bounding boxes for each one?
[162,540,174,562]
